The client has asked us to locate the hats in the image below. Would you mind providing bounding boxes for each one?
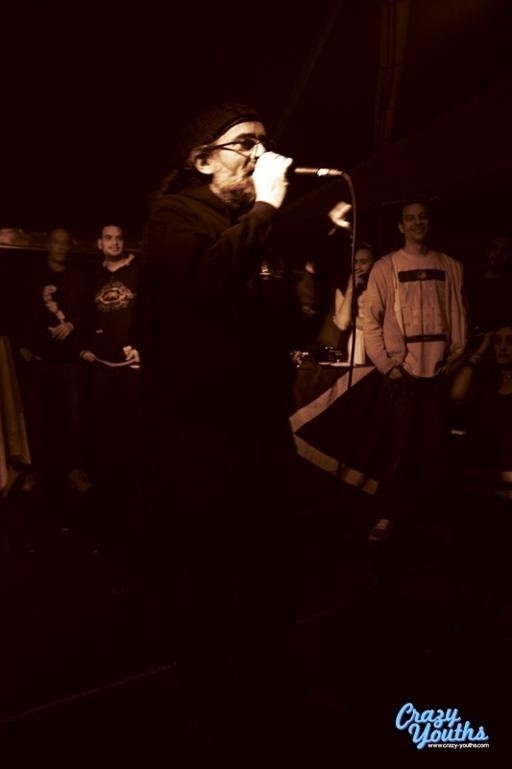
[180,101,261,159]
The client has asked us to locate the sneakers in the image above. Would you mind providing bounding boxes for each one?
[368,516,394,541]
[69,468,94,493]
[22,467,42,492]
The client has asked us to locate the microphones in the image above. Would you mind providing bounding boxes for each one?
[286,165,345,180]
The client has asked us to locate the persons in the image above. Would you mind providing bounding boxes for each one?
[125,95,328,765]
[17,228,95,492]
[70,218,150,503]
[256,196,511,547]
